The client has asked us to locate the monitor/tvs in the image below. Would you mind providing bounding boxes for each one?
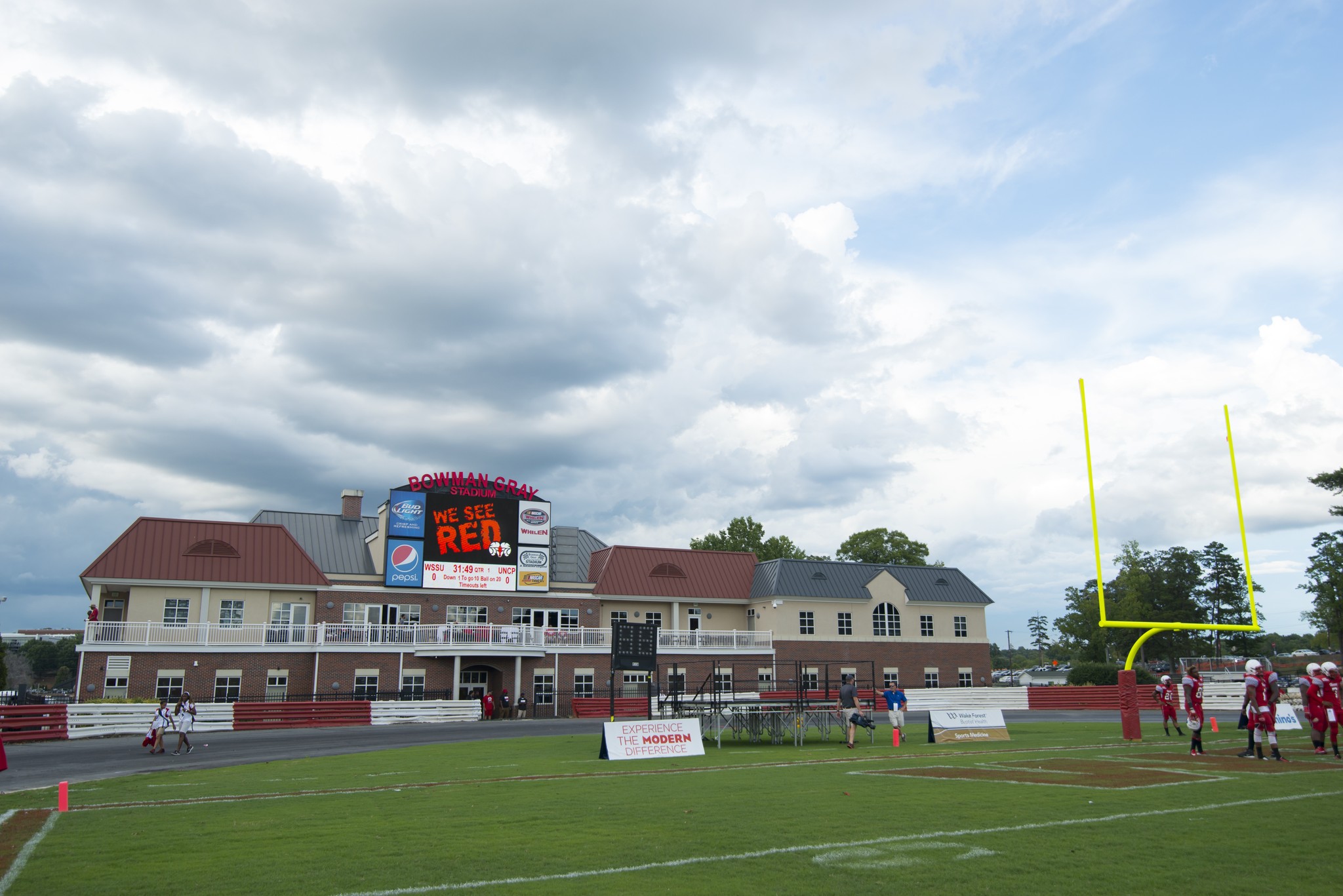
[384,488,551,591]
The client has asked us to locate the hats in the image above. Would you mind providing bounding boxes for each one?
[845,675,855,681]
[521,692,525,695]
[503,689,507,693]
[487,690,493,693]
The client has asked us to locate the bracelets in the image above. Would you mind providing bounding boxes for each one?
[185,709,187,712]
[903,706,905,709]
[837,710,839,712]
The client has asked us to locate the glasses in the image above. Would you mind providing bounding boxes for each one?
[889,685,895,687]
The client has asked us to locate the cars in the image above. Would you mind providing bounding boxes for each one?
[991,648,1340,688]
[30,689,73,695]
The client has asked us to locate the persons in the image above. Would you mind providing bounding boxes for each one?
[1237,670,1280,759]
[464,690,475,700]
[171,691,196,756]
[149,700,176,754]
[1299,662,1328,755]
[1307,661,1343,760]
[1152,676,1186,737]
[499,689,510,719]
[472,690,481,700]
[1243,658,1292,763]
[872,682,907,742]
[482,690,495,720]
[324,614,570,646]
[516,693,528,718]
[1182,666,1207,756]
[836,675,864,749]
[656,689,665,697]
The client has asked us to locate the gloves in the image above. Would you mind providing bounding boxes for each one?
[1189,707,1199,718]
[1257,713,1266,724]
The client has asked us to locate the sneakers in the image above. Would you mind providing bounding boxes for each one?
[1275,756,1293,763]
[1315,746,1328,755]
[1178,733,1186,736]
[1271,751,1277,758]
[171,750,181,755]
[150,748,155,754]
[847,742,855,749]
[1197,751,1209,756]
[1237,747,1255,759]
[186,744,193,754]
[1334,752,1343,760]
[156,749,165,754]
[901,733,906,742]
[1190,749,1198,757]
[1166,732,1171,736]
[1257,755,1270,761]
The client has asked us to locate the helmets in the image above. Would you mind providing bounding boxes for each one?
[1245,659,1263,676]
[1186,715,1201,731]
[1320,661,1339,679]
[1160,675,1171,685]
[1306,662,1322,677]
